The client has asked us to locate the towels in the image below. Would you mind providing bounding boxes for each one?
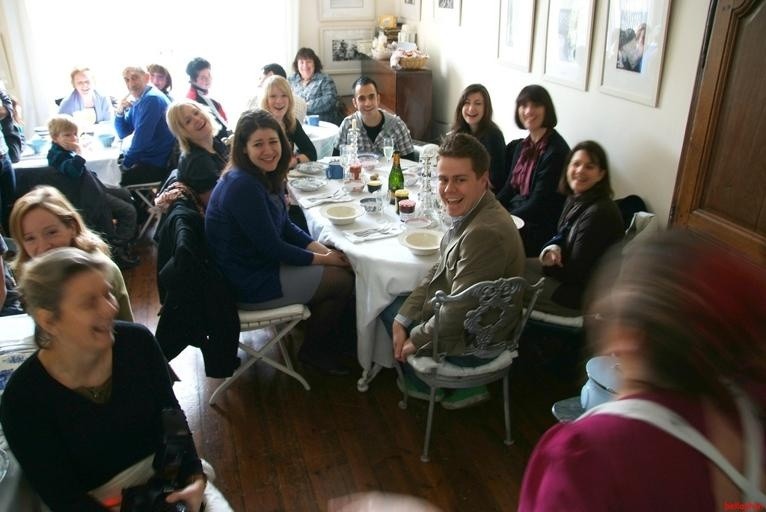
[342,222,404,246]
[297,186,354,210]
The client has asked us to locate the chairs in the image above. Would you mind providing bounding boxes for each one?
[127,182,160,240]
[210,305,312,405]
[398,277,546,464]
[522,211,656,331]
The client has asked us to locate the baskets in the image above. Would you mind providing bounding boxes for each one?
[397,51,429,72]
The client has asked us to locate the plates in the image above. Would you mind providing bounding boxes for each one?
[296,161,329,175]
[290,176,328,191]
[355,152,379,163]
[551,396,588,423]
[510,214,525,230]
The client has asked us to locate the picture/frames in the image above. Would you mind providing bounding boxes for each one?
[496,0,536,73]
[433,0,462,28]
[598,0,671,108]
[318,26,376,74]
[316,0,378,23]
[541,0,596,92]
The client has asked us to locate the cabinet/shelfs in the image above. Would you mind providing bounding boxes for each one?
[360,52,433,142]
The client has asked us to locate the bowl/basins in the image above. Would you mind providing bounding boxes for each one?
[98,134,115,148]
[22,139,47,154]
[403,229,442,256]
[322,205,366,225]
[358,195,383,211]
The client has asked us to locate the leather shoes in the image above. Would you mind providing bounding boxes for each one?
[117,253,142,269]
[296,350,354,377]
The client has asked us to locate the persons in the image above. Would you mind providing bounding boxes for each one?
[146,64,173,99]
[333,77,417,157]
[0,90,21,238]
[448,85,505,187]
[518,230,766,512]
[113,66,180,223]
[285,47,348,126]
[59,66,113,124]
[494,86,571,228]
[184,59,228,127]
[538,142,626,306]
[247,64,307,127]
[164,100,230,207]
[204,110,354,374]
[2,250,208,510]
[9,187,133,330]
[366,135,526,382]
[49,115,142,265]
[256,75,317,171]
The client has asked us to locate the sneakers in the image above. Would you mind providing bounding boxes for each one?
[440,385,493,412]
[395,376,445,406]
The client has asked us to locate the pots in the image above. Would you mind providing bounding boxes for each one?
[581,355,625,411]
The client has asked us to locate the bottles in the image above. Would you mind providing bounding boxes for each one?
[388,151,404,204]
[400,200,415,222]
[395,189,409,214]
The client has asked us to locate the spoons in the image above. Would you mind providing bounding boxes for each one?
[308,188,346,200]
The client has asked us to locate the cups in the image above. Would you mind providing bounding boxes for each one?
[367,181,383,194]
[309,115,320,127]
[404,217,432,229]
[402,172,419,186]
[375,189,390,224]
[342,180,364,195]
[349,161,360,183]
[339,144,354,171]
[383,137,395,172]
